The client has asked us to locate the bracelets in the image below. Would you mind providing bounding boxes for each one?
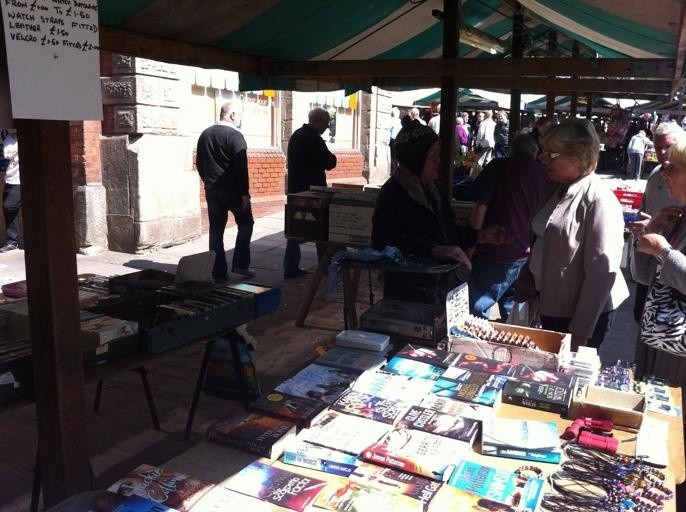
[655,246,675,263]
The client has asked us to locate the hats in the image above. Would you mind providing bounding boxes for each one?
[391,114,437,176]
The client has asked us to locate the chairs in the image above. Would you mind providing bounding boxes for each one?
[340,203,484,349]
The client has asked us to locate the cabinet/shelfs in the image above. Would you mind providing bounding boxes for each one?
[0,246,279,512]
[619,142,659,177]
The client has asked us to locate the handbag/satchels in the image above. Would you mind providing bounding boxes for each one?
[639,219,686,357]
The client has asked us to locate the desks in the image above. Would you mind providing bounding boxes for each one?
[279,233,379,329]
[36,323,684,511]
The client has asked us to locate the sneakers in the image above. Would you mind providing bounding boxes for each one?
[284,270,305,279]
[230,267,256,277]
[214,275,229,283]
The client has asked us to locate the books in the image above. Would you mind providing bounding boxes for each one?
[82,296,604,512]
[449,198,475,227]
[283,175,382,249]
[0,268,283,405]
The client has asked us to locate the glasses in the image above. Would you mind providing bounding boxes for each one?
[542,149,565,159]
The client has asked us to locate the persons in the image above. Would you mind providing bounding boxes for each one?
[281,106,339,280]
[525,116,634,355]
[385,100,657,186]
[468,130,554,323]
[193,101,257,284]
[367,111,478,339]
[0,126,25,254]
[630,131,685,512]
[625,120,686,324]
